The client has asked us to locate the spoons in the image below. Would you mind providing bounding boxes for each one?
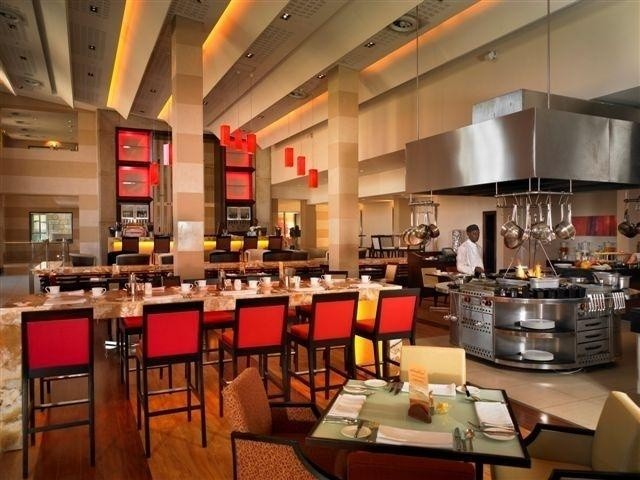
[465,428,475,452]
[471,395,504,403]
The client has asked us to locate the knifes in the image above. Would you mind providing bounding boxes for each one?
[354,418,364,440]
[454,427,467,453]
[387,379,405,397]
[463,384,471,399]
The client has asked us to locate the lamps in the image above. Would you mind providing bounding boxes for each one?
[233,70,243,152]
[296,99,305,175]
[246,73,256,155]
[220,88,231,147]
[285,93,293,167]
[308,95,318,188]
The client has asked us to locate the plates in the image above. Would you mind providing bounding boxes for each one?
[46,295,61,297]
[341,424,371,439]
[345,383,369,393]
[520,318,556,330]
[520,348,555,362]
[483,427,515,442]
[364,379,388,387]
[555,263,572,267]
[456,384,481,396]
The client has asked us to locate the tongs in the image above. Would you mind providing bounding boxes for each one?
[584,290,627,317]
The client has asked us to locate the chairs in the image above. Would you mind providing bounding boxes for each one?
[398,345,467,386]
[490,390,640,480]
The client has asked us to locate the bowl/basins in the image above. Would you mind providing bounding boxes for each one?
[592,266,605,271]
[455,274,474,284]
[568,277,588,283]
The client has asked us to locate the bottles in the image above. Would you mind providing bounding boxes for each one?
[603,240,617,259]
[217,267,226,289]
[576,240,590,257]
[283,267,291,289]
[557,241,569,260]
[128,273,138,295]
[114,221,121,238]
[123,210,146,223]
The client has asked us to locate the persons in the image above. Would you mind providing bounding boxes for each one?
[290,228,295,250]
[456,224,485,277]
[250,218,261,232]
[293,225,301,250]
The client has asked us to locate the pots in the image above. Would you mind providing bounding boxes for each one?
[403,207,440,248]
[617,221,640,238]
[514,265,528,278]
[500,202,577,250]
[612,275,632,289]
[483,272,503,280]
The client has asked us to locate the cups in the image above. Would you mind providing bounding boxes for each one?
[321,274,332,282]
[360,275,372,283]
[577,255,592,268]
[511,287,517,297]
[44,285,61,295]
[310,277,321,285]
[223,229,228,236]
[91,287,106,296]
[143,276,302,294]
[499,288,506,296]
